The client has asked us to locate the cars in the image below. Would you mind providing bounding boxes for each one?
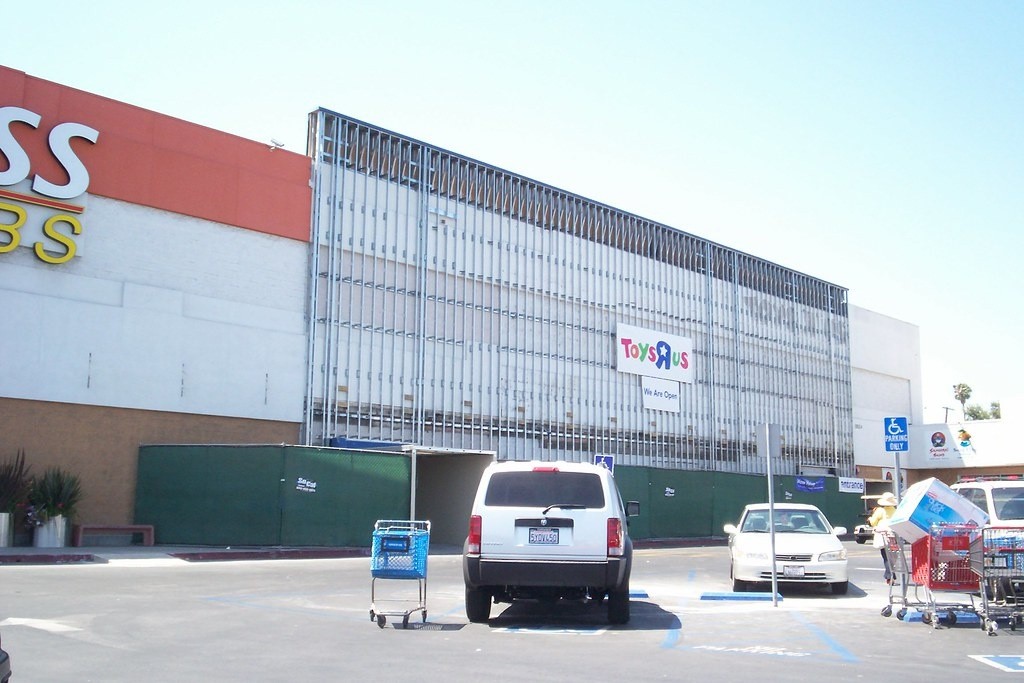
[725,500,849,595]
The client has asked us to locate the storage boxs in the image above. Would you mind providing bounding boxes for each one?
[888,477,991,562]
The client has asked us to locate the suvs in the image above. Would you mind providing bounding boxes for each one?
[461,458,640,624]
[853,517,876,544]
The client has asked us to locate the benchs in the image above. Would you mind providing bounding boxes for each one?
[73,525,155,546]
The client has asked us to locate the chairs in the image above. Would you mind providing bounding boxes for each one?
[750,518,767,531]
[791,515,809,529]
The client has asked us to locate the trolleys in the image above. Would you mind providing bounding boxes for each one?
[872,521,1024,637]
[368,519,432,630]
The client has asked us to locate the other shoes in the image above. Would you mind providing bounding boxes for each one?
[887,579,894,584]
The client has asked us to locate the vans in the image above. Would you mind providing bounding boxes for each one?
[949,474,1024,543]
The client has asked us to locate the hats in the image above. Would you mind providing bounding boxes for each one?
[877,492,898,506]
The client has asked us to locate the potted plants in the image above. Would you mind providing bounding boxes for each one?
[0,447,46,548]
[26,463,87,547]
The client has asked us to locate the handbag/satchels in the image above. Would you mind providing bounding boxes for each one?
[873,507,890,550]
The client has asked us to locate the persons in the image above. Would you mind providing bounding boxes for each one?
[867,492,898,584]
